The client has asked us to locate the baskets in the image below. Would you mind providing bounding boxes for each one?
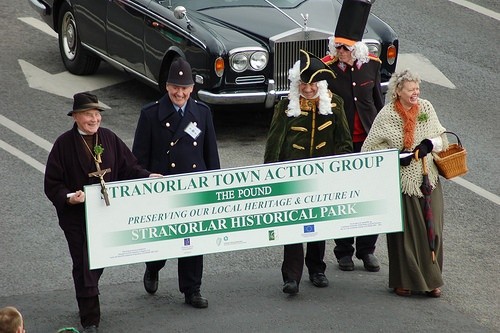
[431,131,470,180]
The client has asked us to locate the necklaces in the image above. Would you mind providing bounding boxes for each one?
[80,133,98,158]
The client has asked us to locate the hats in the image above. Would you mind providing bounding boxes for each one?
[166,57,195,86]
[299,49,336,84]
[334,0,372,47]
[67,93,105,116]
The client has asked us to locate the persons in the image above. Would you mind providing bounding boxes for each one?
[132,58,221,308]
[44,92,163,333]
[56,326,80,333]
[320,0,384,272]
[361,70,449,298]
[264,49,353,294]
[0,305,26,333]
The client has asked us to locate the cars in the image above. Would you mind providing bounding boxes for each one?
[26,0,400,125]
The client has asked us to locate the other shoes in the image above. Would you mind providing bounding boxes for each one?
[337,255,355,271]
[425,287,441,297]
[185,291,208,308]
[355,252,380,272]
[309,272,329,288]
[283,279,299,293]
[80,325,98,333]
[394,288,412,297]
[144,270,159,295]
